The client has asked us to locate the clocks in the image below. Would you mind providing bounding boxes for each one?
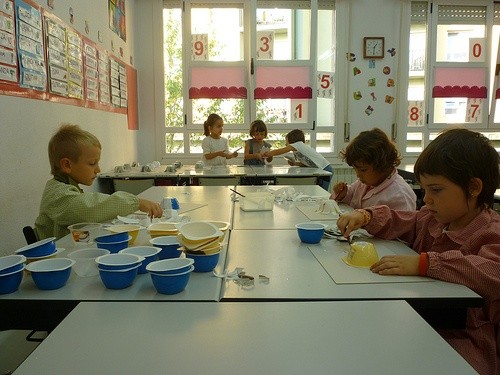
[363,37,384,58]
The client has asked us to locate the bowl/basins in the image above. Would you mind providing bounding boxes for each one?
[118,246,162,274]
[93,230,133,254]
[113,160,183,173]
[109,222,143,245]
[296,223,326,244]
[159,197,182,211]
[25,256,76,290]
[67,222,103,246]
[145,257,195,295]
[0,254,26,294]
[94,252,142,290]
[13,236,66,266]
[342,241,379,268]
[67,247,111,278]
[146,221,230,272]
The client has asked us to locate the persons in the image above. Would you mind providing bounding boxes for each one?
[200,113,238,165]
[34,122,163,242]
[329,128,417,212]
[336,129,500,375]
[261,129,333,191]
[243,120,272,166]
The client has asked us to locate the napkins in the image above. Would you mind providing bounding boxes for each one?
[297,205,344,221]
[174,202,209,216]
[308,244,436,285]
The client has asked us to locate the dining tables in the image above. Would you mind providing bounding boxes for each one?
[0,186,500,375]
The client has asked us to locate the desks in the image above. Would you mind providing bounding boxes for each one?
[101,163,333,196]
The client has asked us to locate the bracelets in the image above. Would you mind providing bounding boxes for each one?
[264,151,268,157]
[354,209,370,227]
[420,252,427,277]
